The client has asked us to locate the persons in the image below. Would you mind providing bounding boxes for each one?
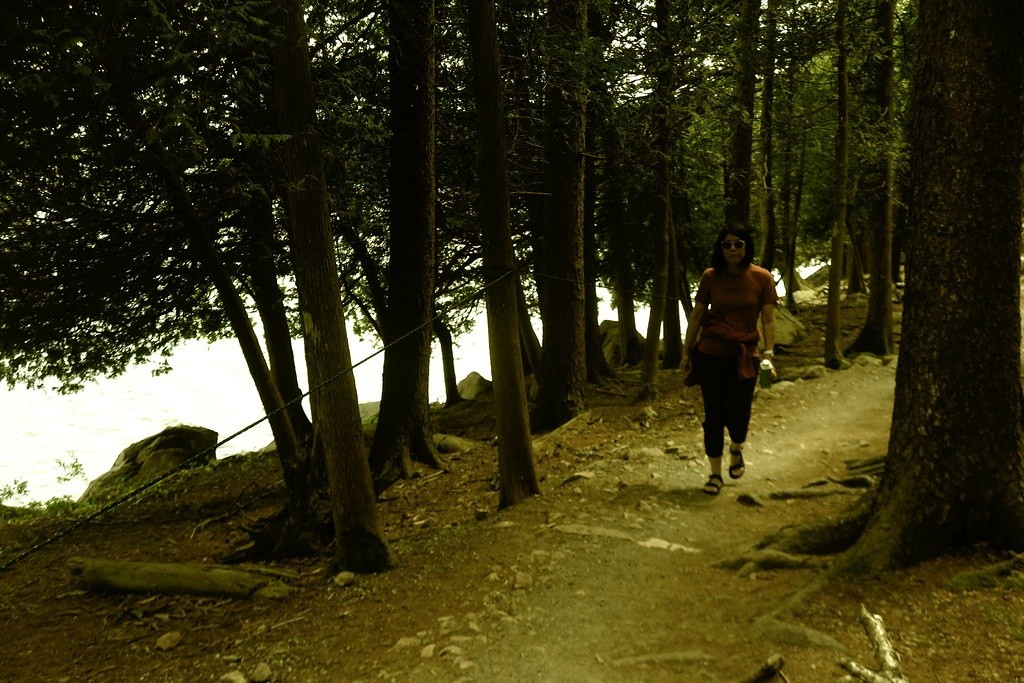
[678,221,779,495]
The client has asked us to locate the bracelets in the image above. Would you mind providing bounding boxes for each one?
[764,349,774,357]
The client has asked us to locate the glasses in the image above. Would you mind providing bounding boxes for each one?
[722,241,745,249]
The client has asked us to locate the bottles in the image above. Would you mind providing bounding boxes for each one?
[759,359,772,389]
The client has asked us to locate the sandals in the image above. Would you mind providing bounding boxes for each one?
[704,474,722,494]
[729,444,745,478]
